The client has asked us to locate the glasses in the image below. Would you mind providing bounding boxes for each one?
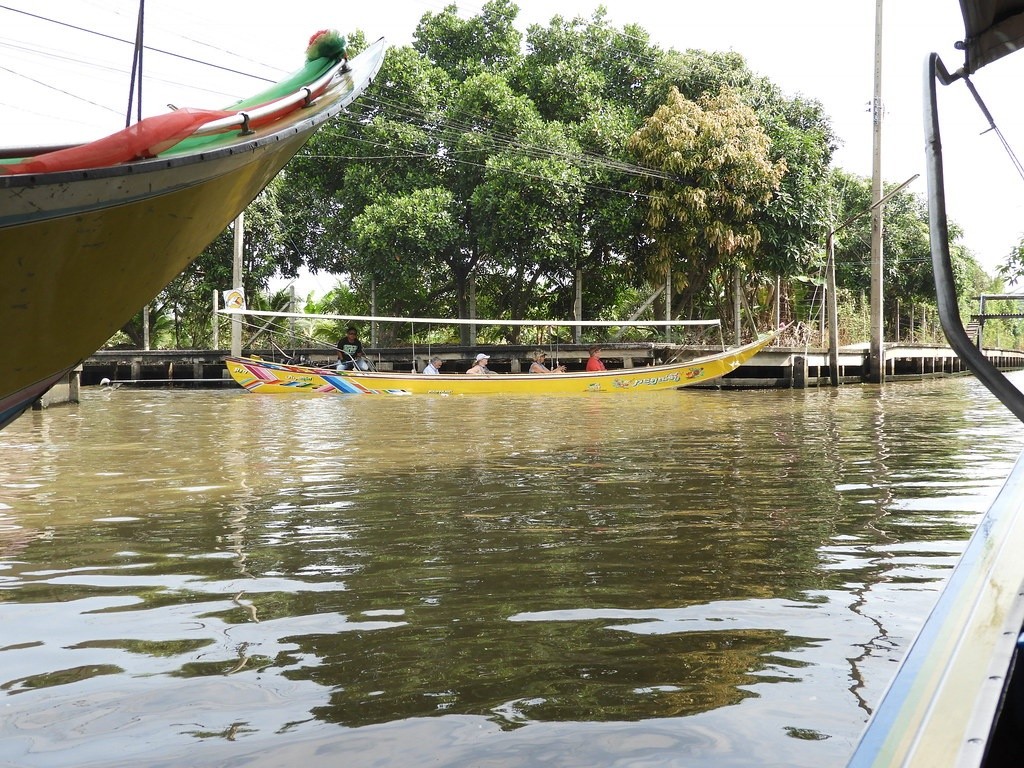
[349,332,356,335]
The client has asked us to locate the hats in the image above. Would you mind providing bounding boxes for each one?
[476,354,490,361]
[533,349,546,358]
[589,345,603,355]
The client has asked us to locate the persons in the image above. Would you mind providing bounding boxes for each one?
[336,327,368,371]
[422,357,443,374]
[466,353,498,374]
[529,348,566,373]
[586,347,607,371]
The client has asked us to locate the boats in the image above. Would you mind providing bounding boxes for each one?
[207,306,796,398]
[0,26,391,437]
